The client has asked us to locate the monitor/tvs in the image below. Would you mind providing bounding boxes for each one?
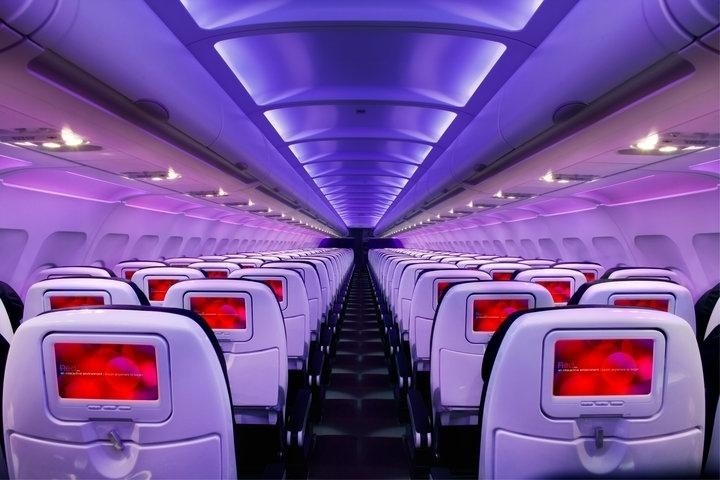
[207,270,223,281]
[43,333,171,423]
[248,278,288,310]
[44,290,111,310]
[530,276,573,305]
[493,268,513,280]
[432,277,473,309]
[579,269,597,284]
[143,274,186,303]
[465,293,534,342]
[122,268,134,281]
[540,330,666,421]
[183,293,252,341]
[610,295,673,315]
